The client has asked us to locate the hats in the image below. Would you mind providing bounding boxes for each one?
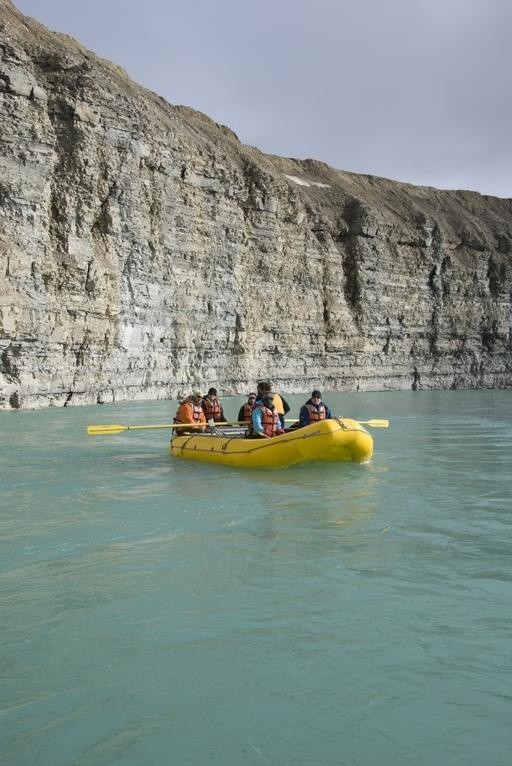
[312,391,321,397]
[193,389,203,398]
[248,392,256,399]
[263,393,274,400]
[208,388,217,396]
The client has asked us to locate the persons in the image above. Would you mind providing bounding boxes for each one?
[174,381,333,439]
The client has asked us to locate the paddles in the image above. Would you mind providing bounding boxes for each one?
[87,422,233,435]
[234,419,389,427]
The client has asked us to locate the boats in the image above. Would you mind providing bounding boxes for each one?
[165,418,377,471]
